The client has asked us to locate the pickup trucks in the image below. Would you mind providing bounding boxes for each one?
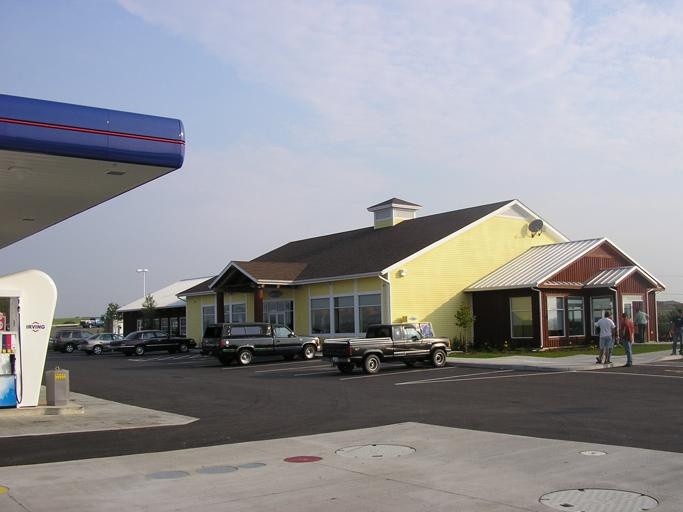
[81,317,105,328]
[322,324,450,374]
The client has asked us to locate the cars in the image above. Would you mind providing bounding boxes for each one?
[53,329,197,356]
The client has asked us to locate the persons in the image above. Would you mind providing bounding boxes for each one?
[671,310,683,355]
[622,313,634,367]
[597,311,616,364]
[634,309,647,343]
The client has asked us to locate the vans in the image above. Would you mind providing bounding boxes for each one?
[202,323,320,367]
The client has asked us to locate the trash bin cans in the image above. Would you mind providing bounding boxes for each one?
[45,370,69,406]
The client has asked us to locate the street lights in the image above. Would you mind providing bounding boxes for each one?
[136,268,148,297]
